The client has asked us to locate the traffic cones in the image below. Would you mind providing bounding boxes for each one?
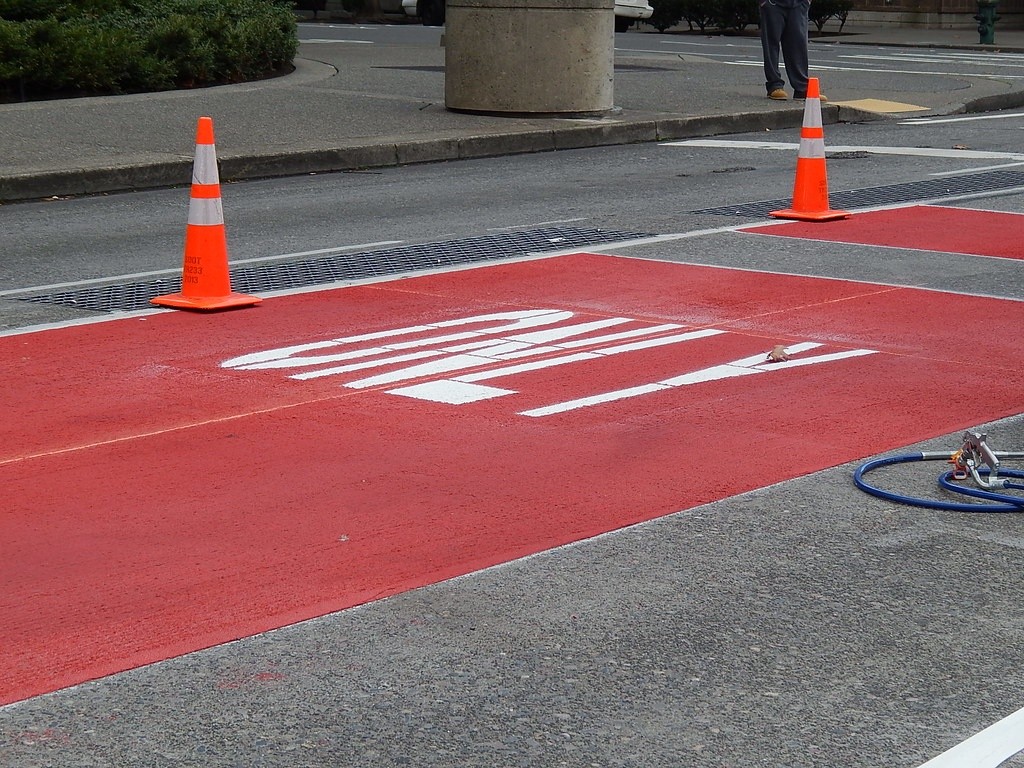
[149,115,264,313]
[769,77,854,222]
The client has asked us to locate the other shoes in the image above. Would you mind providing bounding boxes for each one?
[794,93,827,102]
[767,89,788,100]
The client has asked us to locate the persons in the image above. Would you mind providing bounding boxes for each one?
[755,0,828,102]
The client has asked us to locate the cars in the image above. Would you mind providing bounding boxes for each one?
[404,0,654,33]
